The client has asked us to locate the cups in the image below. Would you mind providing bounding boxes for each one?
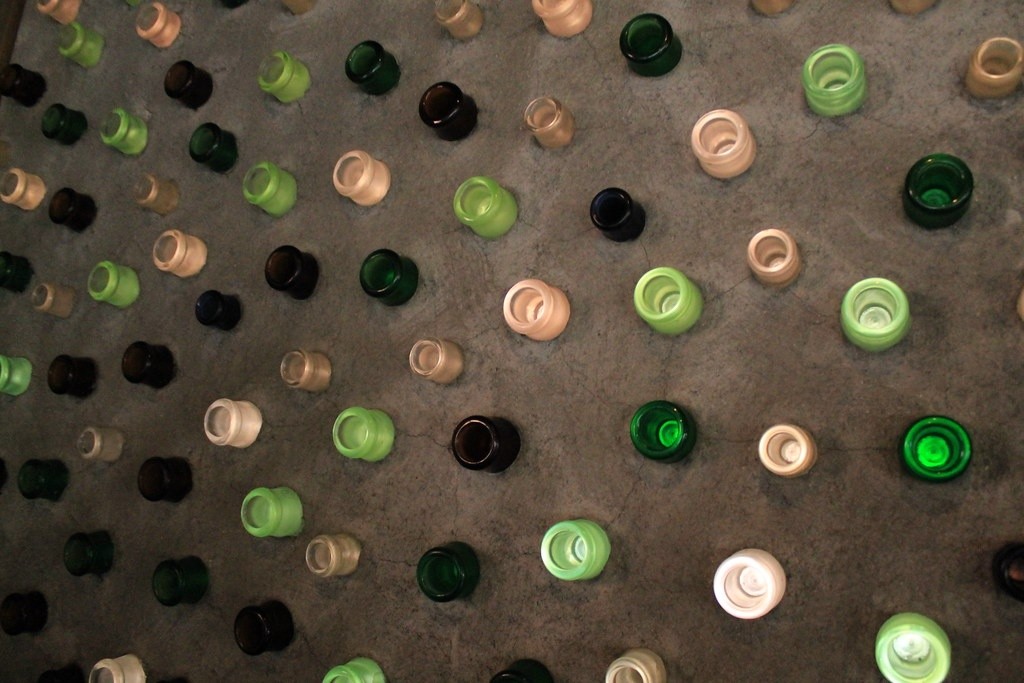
[89,653,146,683]
[714,549,786,619]
[0,0,1023,608]
[873,613,951,683]
[36,663,86,683]
[604,650,667,683]
[1,591,48,634]
[233,603,295,656]
[321,656,388,683]
[488,659,554,683]
